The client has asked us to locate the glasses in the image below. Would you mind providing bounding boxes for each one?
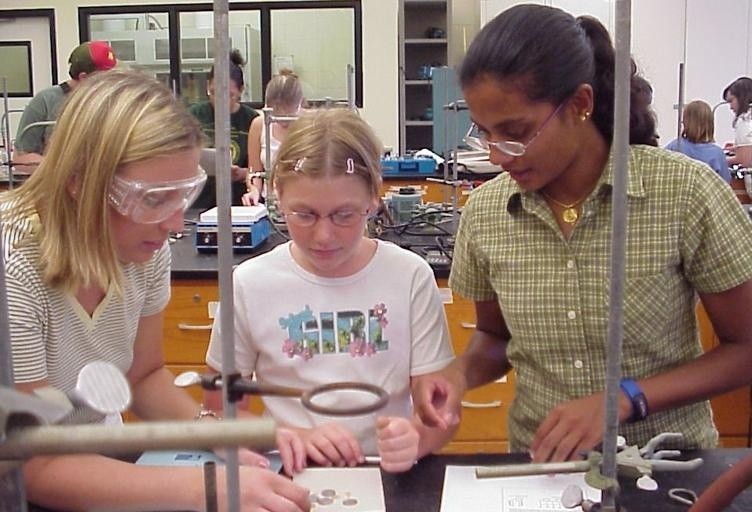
[206,81,242,99]
[282,209,368,227]
[271,100,301,122]
[108,166,207,225]
[463,101,566,156]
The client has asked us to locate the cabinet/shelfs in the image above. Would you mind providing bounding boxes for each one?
[398,0,482,158]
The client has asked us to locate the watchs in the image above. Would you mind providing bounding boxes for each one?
[618,376,650,425]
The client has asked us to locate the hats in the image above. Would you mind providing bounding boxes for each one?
[69,42,117,77]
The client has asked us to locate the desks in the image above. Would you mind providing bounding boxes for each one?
[126,177,751,435]
[30,444,751,512]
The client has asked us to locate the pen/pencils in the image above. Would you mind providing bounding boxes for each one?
[364,455,417,467]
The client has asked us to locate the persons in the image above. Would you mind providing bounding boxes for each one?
[203,113,461,474]
[239,69,311,209]
[410,4,752,476]
[11,40,118,175]
[660,100,732,190]
[185,52,260,212]
[721,77,752,200]
[0,72,311,512]
